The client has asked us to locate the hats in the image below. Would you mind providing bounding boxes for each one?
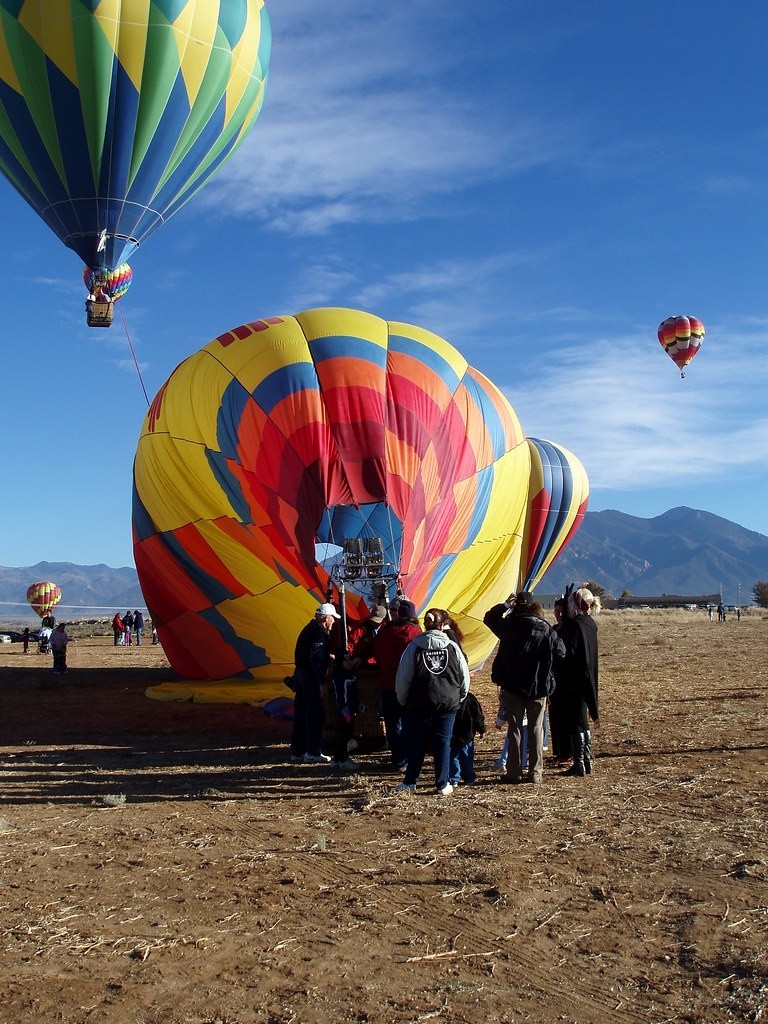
[398,600,415,617]
[315,603,341,619]
[389,594,411,608]
[513,591,533,605]
[368,605,386,624]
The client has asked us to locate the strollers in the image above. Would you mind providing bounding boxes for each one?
[37,626,53,654]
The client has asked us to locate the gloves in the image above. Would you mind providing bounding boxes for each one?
[342,706,351,722]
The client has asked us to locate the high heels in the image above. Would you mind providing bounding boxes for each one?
[562,762,584,776]
[584,759,592,773]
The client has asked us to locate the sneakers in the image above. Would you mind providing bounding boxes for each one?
[396,782,416,790]
[438,782,453,795]
[305,750,331,763]
[291,755,303,763]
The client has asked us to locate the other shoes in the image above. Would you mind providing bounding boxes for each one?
[531,772,542,783]
[499,774,522,784]
[63,670,70,674]
[451,782,458,787]
[54,669,61,675]
[465,778,474,783]
[332,758,358,769]
[546,756,574,767]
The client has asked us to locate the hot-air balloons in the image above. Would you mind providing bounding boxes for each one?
[131,307,532,756]
[657,314,706,378]
[0,0,272,328]
[83,261,133,302]
[26,581,63,618]
[516,436,589,593]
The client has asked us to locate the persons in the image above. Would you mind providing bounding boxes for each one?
[448,692,487,787]
[328,593,470,797]
[483,582,600,786]
[21,628,33,653]
[40,610,55,654]
[708,603,741,622]
[50,623,75,674]
[113,611,160,646]
[289,602,356,762]
